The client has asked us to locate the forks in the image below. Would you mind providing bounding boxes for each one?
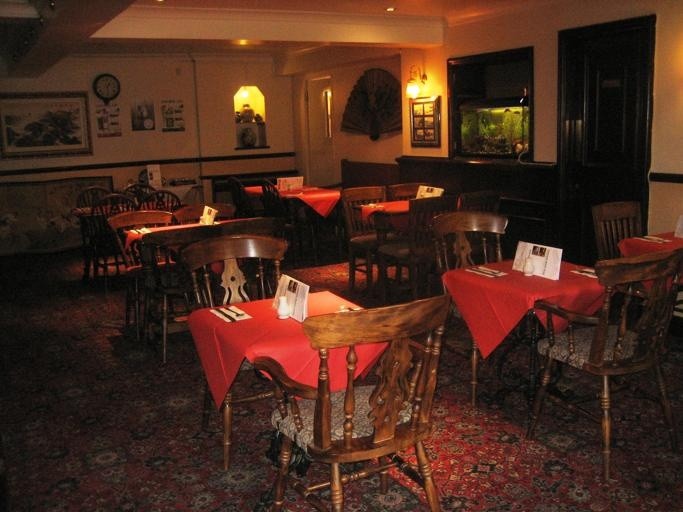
[472,266,501,274]
[224,304,243,317]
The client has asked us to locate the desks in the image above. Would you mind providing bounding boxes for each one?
[617,228,683,294]
[440,256,617,416]
[180,285,409,488]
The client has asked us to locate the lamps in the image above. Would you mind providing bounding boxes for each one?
[407,62,426,98]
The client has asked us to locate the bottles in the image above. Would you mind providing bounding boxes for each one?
[521,256,533,277]
[275,293,292,321]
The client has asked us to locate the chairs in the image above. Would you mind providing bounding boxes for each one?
[70,177,552,362]
[270,292,455,505]
[590,198,665,377]
[518,246,680,489]
[173,230,301,471]
[423,209,547,412]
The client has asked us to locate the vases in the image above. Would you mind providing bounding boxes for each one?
[241,103,254,122]
[239,127,257,147]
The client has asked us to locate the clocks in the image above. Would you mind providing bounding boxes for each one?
[93,73,120,105]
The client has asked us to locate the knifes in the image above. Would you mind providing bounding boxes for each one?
[214,308,236,324]
[467,268,499,278]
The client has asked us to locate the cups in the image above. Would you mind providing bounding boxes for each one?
[199,216,205,224]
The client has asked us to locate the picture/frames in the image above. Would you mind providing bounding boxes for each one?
[408,95,441,147]
[0,91,92,157]
[0,175,114,258]
